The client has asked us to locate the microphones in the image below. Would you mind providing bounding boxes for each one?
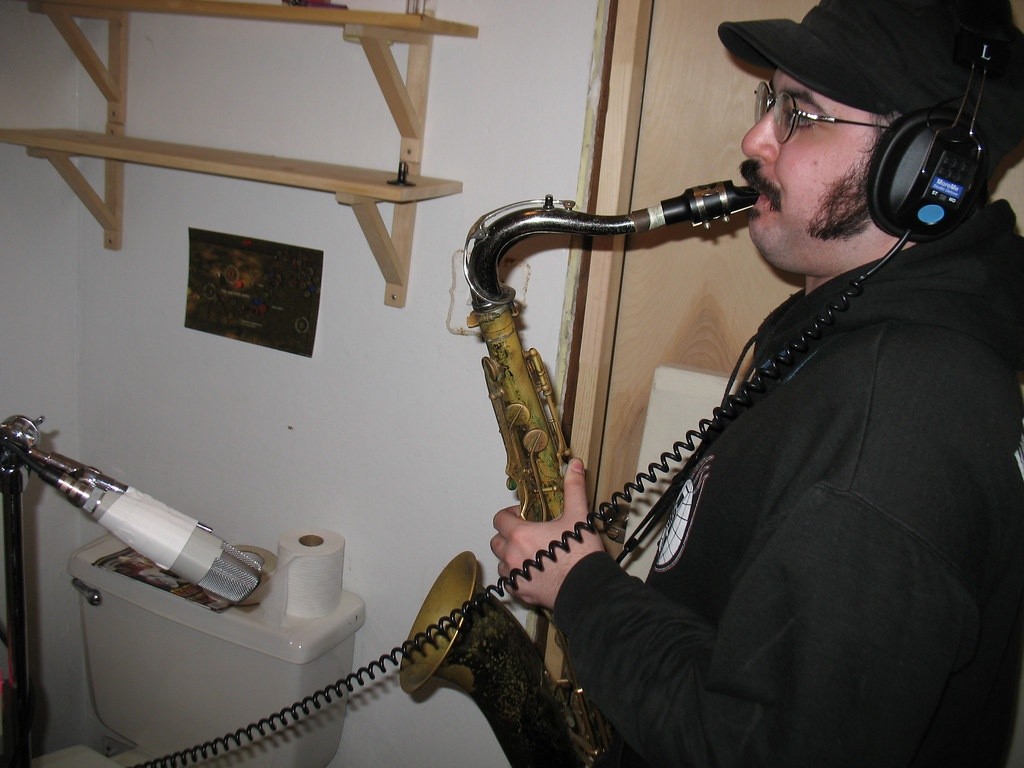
[37,466,260,602]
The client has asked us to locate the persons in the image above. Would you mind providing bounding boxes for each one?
[490,0,1024,768]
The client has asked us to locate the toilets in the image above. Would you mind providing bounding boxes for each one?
[33,530,366,766]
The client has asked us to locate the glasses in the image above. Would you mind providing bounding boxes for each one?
[754,81,891,145]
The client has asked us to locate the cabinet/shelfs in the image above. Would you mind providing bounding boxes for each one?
[0,0,482,307]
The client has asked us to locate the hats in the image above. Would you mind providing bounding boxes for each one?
[718,0,1024,181]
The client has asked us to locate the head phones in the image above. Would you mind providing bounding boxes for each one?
[865,0,1011,244]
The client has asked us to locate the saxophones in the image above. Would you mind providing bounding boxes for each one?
[395,179,764,768]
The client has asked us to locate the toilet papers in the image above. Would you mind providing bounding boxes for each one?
[261,527,346,617]
